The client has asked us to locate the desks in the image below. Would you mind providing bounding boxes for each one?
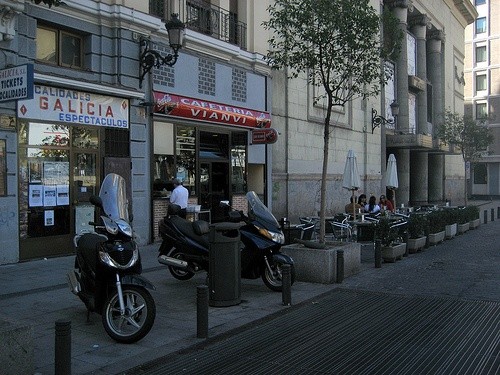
[310,216,334,220]
[290,224,305,227]
[190,210,212,223]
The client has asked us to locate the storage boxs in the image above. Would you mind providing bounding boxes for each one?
[76,204,94,234]
[187,205,202,212]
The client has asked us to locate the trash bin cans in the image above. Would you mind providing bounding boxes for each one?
[208,222,241,307]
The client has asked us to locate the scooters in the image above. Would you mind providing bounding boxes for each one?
[70,174,158,343]
[158,192,294,290]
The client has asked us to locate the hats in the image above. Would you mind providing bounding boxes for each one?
[380,195,385,199]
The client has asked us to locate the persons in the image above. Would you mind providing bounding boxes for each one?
[169,179,189,220]
[345,194,393,219]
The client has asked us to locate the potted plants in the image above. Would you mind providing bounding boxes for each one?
[372,205,481,264]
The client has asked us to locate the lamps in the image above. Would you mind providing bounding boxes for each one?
[137,13,186,89]
[372,99,400,133]
[139,101,159,106]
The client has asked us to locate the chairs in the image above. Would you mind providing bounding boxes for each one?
[279,205,447,246]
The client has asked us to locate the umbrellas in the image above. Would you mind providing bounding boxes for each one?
[384,154,399,214]
[342,150,361,221]
